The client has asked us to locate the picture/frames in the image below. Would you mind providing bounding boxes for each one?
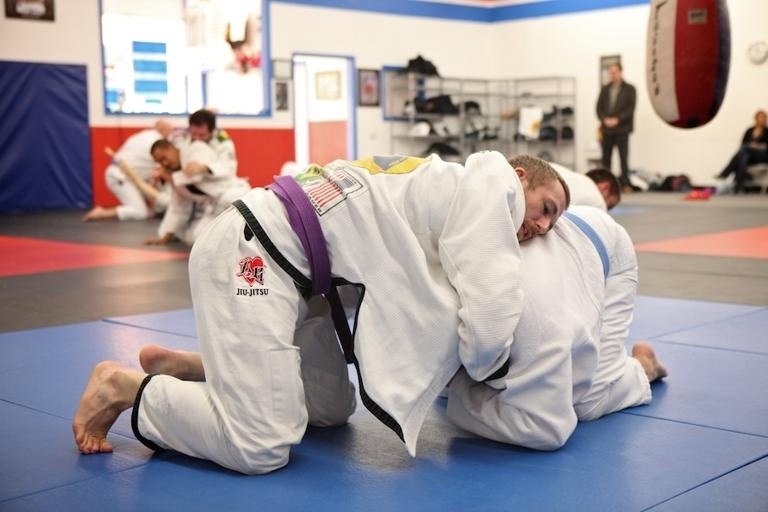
[358,68,381,106]
[275,82,289,111]
[382,66,425,121]
[600,55,621,92]
[272,59,293,80]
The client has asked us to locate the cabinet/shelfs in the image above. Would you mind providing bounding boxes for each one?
[389,72,577,171]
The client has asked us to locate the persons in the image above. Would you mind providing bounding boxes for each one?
[548,163,622,215]
[79,150,572,475]
[718,112,768,194]
[86,111,252,248]
[443,204,666,452]
[597,63,636,192]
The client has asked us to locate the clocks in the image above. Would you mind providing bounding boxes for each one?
[747,41,768,65]
